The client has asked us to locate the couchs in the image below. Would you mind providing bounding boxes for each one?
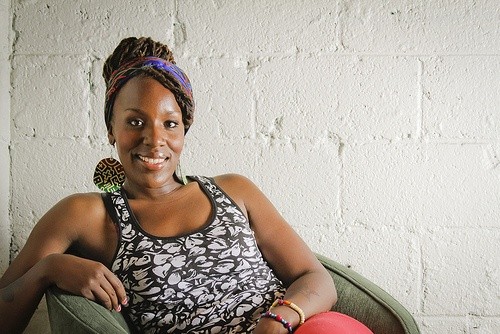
[45,250,422,334]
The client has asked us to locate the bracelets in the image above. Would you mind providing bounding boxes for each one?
[269,298,305,324]
[260,312,292,334]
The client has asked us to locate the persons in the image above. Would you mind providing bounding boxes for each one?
[0,36,375,334]
